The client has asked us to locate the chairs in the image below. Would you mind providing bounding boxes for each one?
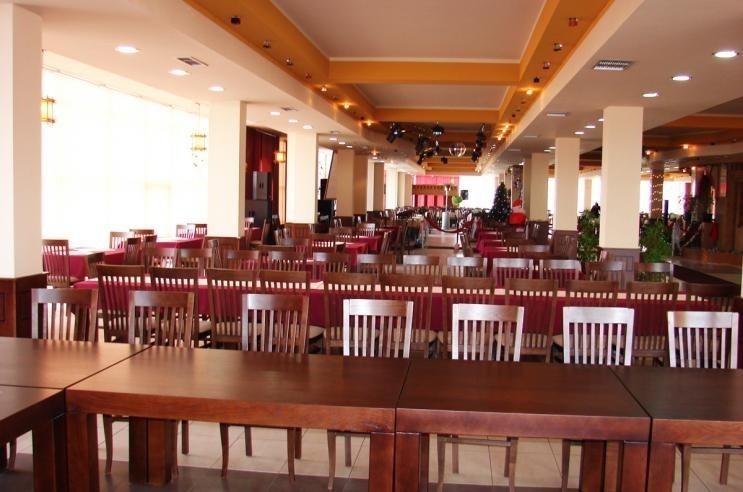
[0,205,743,491]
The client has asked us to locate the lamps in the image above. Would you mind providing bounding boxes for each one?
[188,104,206,167]
[271,137,288,166]
[40,95,56,129]
[382,118,489,172]
